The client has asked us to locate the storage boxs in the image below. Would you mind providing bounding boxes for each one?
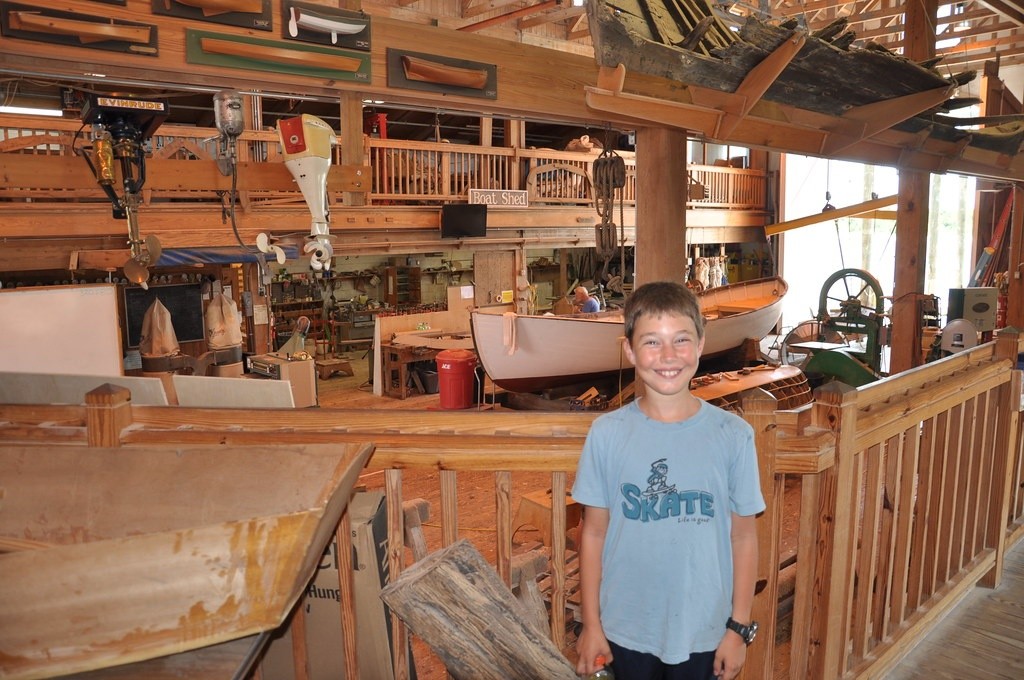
[259,490,419,680]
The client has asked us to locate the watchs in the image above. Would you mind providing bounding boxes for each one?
[726,618,759,644]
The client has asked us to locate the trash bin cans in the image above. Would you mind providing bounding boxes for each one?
[434,348,478,409]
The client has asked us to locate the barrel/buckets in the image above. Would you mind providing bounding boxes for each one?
[435,348,478,410]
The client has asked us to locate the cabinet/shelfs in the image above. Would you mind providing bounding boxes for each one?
[270,282,323,351]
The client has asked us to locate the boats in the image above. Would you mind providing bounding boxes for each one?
[469,276,788,395]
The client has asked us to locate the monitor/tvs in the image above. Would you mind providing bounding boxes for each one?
[441,204,487,237]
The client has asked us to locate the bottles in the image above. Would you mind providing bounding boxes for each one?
[581,654,615,680]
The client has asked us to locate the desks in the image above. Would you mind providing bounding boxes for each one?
[379,344,442,400]
[511,487,584,547]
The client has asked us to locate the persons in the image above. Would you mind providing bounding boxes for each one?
[574,286,599,312]
[570,282,767,680]
